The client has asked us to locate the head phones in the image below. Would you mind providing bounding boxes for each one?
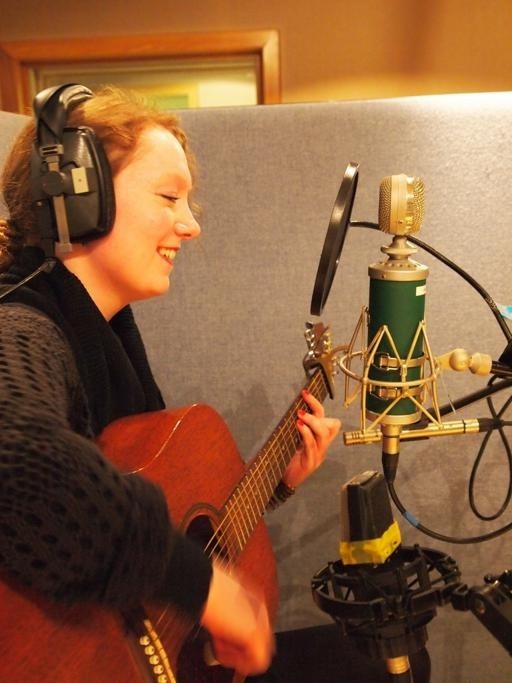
[28,85,118,248]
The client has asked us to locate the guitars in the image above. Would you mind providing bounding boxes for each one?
[1,322,346,682]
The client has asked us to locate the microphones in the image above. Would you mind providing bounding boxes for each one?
[341,416,501,444]
[365,171,424,427]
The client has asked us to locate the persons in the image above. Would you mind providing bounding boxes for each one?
[0,85,432,682]
